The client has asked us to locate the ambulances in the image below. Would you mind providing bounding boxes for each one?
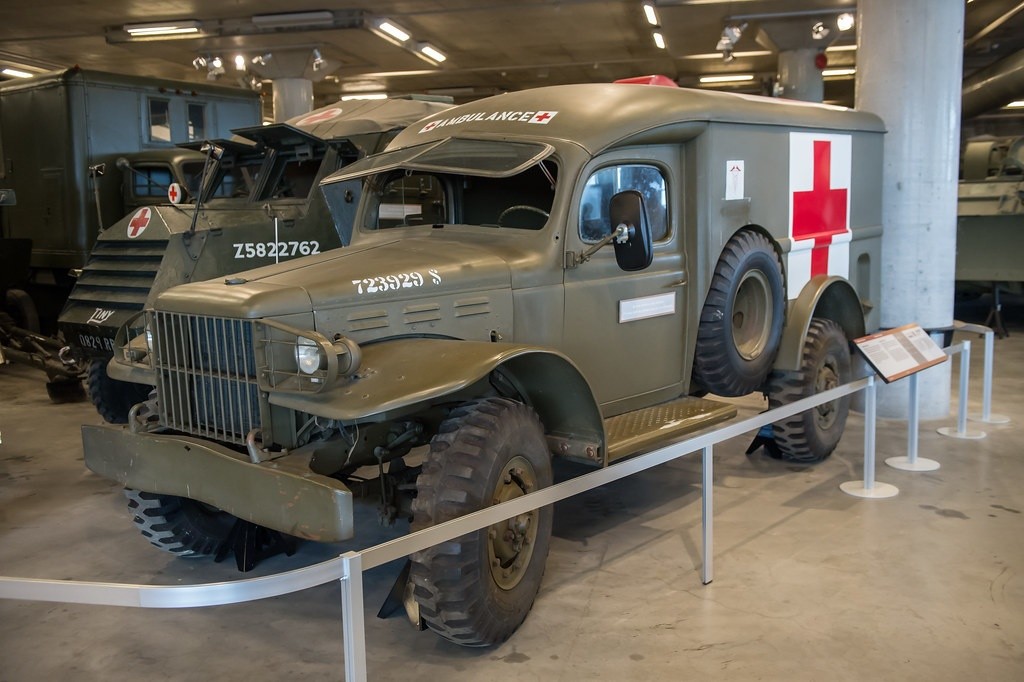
[1,65,890,652]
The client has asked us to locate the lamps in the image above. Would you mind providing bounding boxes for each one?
[261,52,272,65]
[812,22,831,40]
[715,30,734,52]
[837,12,855,32]
[252,12,334,28]
[416,42,449,63]
[199,55,214,67]
[213,55,224,68]
[725,21,749,43]
[312,48,322,61]
[122,20,202,36]
[374,17,413,42]
